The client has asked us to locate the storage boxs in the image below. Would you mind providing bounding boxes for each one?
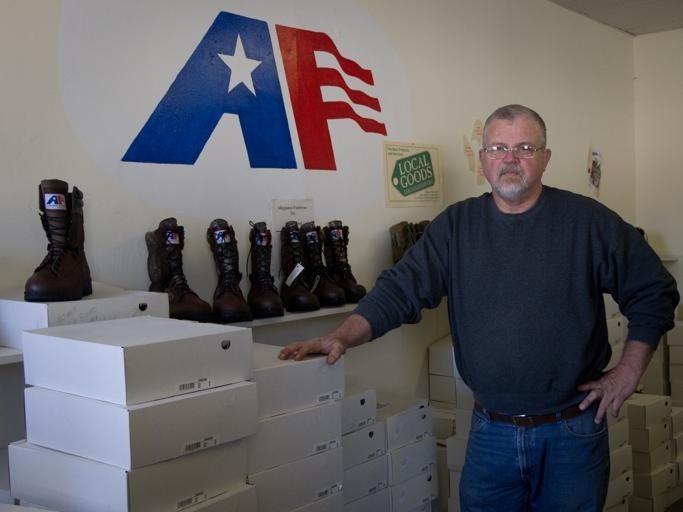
[392,464,441,506]
[388,434,439,487]
[21,385,257,468]
[433,406,464,441]
[669,344,681,361]
[642,466,677,500]
[251,402,348,471]
[608,446,638,479]
[608,422,634,449]
[628,421,673,452]
[667,361,683,381]
[250,442,350,504]
[8,439,258,507]
[22,314,256,406]
[431,371,464,405]
[671,381,683,404]
[2,277,169,351]
[425,333,465,379]
[666,322,683,345]
[248,336,350,418]
[347,454,392,500]
[624,395,673,426]
[338,418,394,468]
[635,444,678,472]
[610,472,639,506]
[378,396,435,450]
[339,388,380,438]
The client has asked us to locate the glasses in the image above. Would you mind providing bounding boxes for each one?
[482,143,547,161]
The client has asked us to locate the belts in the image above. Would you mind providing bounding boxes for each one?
[472,401,594,430]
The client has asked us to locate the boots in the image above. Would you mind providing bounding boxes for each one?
[277,222,321,313]
[144,218,212,323]
[299,222,347,308]
[206,219,254,325]
[388,220,417,265]
[323,220,367,304]
[243,222,284,320]
[23,179,94,304]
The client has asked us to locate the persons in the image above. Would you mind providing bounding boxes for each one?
[279,105,680,512]
